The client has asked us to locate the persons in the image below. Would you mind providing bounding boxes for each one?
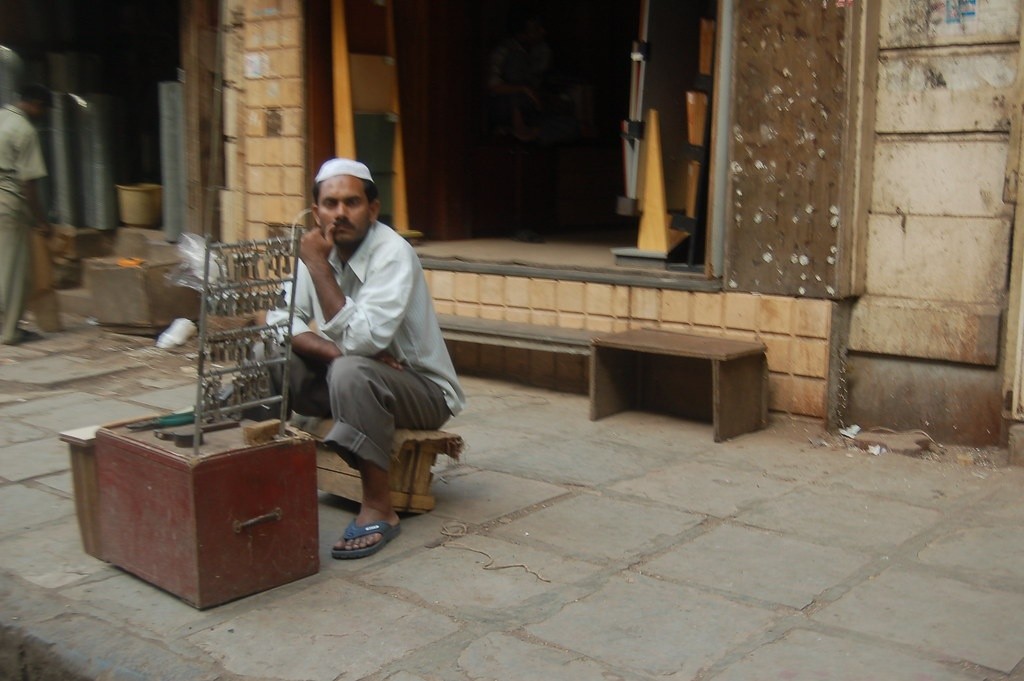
[0,45,49,345]
[483,6,600,143]
[235,159,465,561]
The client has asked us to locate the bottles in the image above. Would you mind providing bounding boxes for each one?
[158,319,195,349]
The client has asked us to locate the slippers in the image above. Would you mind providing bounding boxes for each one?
[331,517,400,559]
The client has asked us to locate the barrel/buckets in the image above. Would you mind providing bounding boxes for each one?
[115,181,163,227]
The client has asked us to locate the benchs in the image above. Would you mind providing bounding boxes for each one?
[586,326,771,445]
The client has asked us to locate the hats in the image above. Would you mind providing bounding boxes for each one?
[315,158,374,183]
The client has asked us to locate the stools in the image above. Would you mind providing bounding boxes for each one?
[300,412,464,516]
